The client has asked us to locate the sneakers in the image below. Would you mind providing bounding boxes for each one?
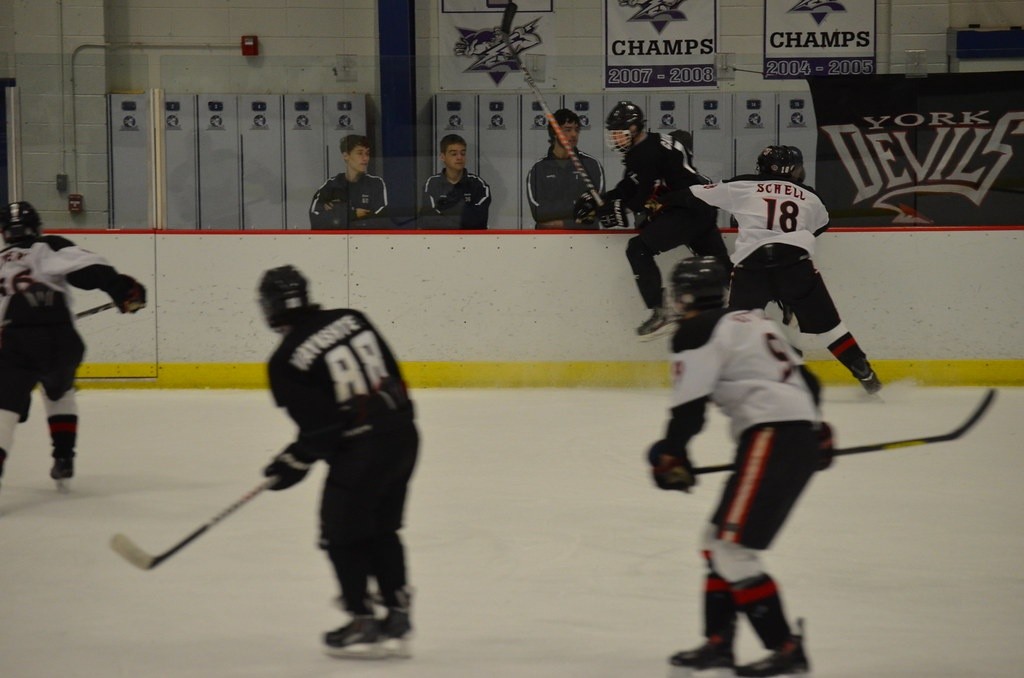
[635,307,680,342]
[49,452,74,494]
[861,369,889,408]
[731,645,811,678]
[377,604,419,658]
[668,642,735,675]
[319,616,386,658]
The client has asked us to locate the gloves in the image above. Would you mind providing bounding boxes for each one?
[109,276,149,314]
[813,421,836,470]
[648,439,697,494]
[572,193,631,230]
[263,442,310,492]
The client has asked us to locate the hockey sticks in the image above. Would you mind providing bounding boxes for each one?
[686,389,998,474]
[108,477,280,571]
[71,302,115,321]
[498,2,604,206]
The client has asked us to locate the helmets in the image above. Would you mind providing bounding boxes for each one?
[256,263,310,317]
[602,100,647,156]
[755,145,797,178]
[671,255,727,308]
[0,200,41,230]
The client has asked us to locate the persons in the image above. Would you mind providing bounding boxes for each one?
[526,108,605,229]
[417,134,491,230]
[309,134,392,229]
[257,266,420,661]
[633,146,886,403]
[647,256,833,678]
[572,101,735,343]
[0,201,147,494]
[634,130,713,228]
